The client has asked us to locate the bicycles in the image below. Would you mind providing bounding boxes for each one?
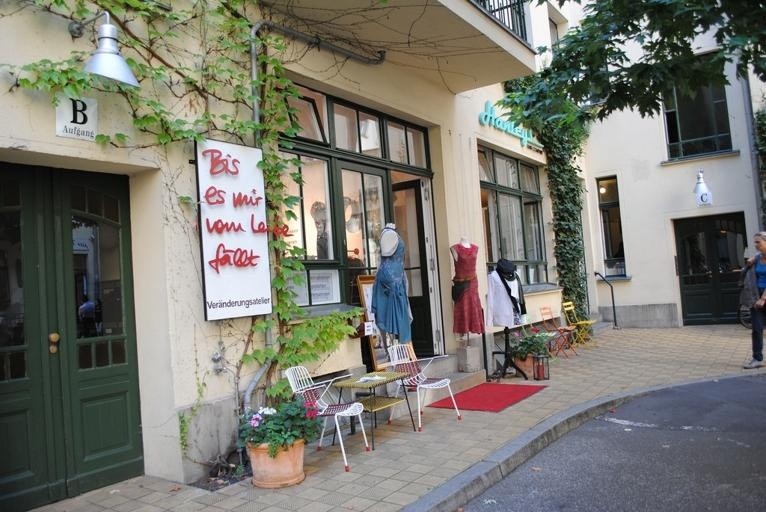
[738,300,753,329]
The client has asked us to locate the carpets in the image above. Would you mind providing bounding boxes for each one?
[425,383,544,413]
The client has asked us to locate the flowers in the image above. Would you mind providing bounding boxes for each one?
[511,328,550,361]
[236,397,324,458]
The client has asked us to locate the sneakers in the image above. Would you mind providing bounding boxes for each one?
[744,359,764,368]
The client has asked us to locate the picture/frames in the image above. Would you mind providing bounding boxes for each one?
[357,275,411,371]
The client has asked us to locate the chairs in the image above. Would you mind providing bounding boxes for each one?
[522,302,596,364]
[388,344,462,433]
[285,366,371,472]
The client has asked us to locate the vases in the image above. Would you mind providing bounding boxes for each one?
[246,439,306,489]
[514,354,534,378]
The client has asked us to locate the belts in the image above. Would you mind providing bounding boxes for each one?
[453,275,477,281]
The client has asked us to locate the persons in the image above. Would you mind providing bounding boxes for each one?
[372,223,411,344]
[741,231,766,369]
[449,237,485,336]
[488,258,527,327]
[79,294,98,336]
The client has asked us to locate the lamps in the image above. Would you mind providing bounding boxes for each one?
[69,11,140,88]
[692,168,709,193]
